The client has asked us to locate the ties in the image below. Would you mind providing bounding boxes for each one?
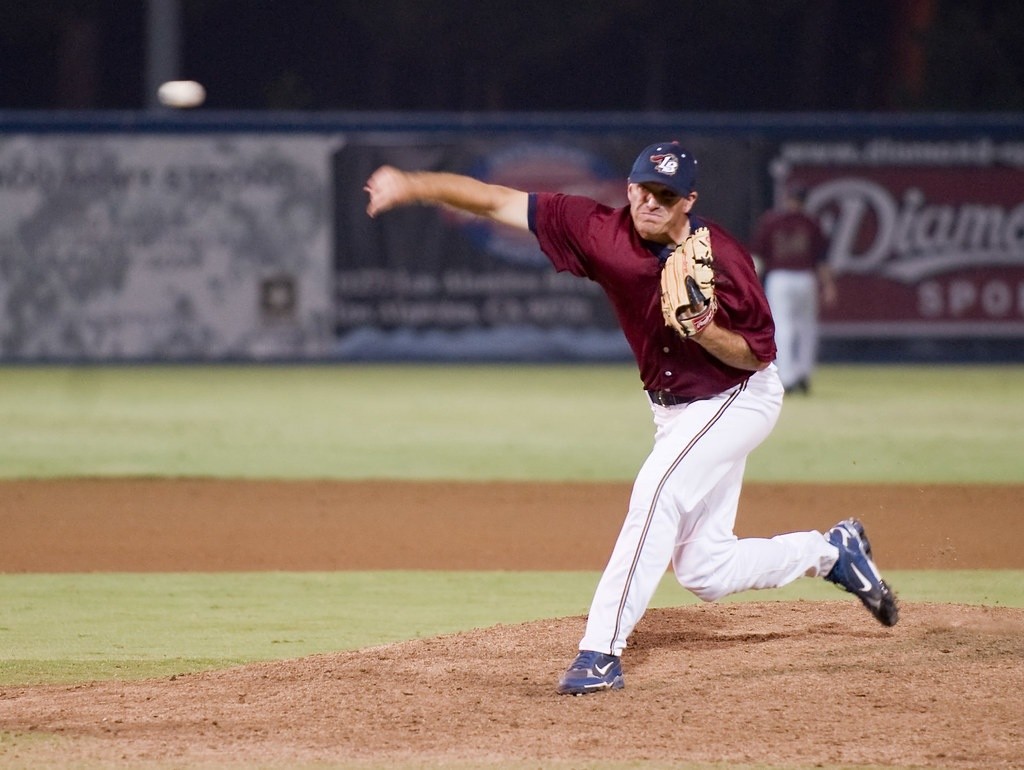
[629,141,698,199]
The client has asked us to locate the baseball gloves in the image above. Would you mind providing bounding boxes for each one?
[659,226,719,339]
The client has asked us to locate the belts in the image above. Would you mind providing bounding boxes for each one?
[649,388,696,409]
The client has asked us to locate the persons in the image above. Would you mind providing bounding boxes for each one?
[360,139,898,694]
[754,181,837,398]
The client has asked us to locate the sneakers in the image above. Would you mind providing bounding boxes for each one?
[823,517,899,626]
[559,651,625,695]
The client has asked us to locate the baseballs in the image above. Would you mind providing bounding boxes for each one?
[158,80,205,109]
